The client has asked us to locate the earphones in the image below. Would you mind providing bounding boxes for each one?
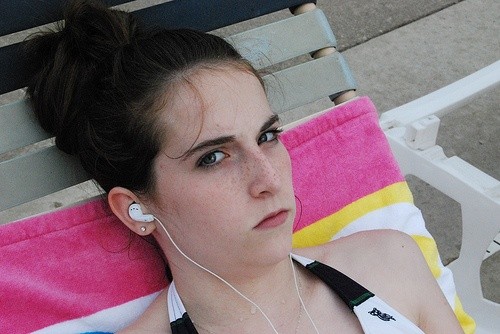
[127,202,155,224]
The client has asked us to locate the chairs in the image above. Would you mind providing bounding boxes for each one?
[0,0,475,334]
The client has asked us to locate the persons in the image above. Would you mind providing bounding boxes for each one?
[20,0,469,334]
